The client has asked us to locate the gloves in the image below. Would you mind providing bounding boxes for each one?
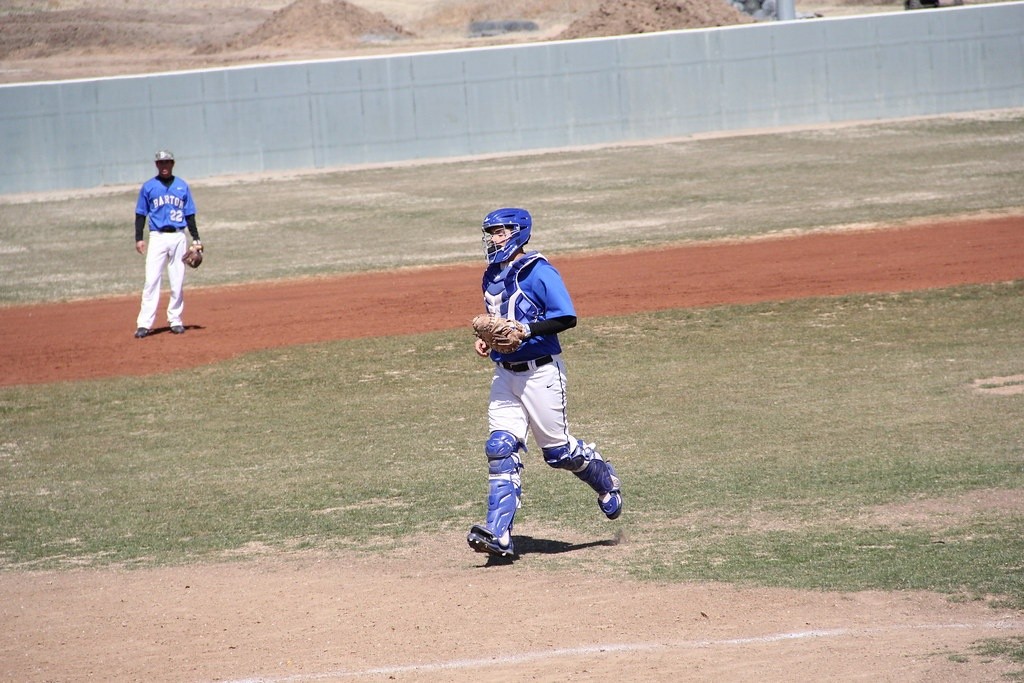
[182,244,203,268]
[472,314,526,354]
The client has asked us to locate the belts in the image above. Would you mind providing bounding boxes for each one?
[160,226,186,232]
[496,355,553,372]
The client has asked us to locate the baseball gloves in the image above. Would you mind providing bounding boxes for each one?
[472,314,525,355]
[182,244,203,268]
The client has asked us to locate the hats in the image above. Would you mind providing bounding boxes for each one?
[154,149,173,161]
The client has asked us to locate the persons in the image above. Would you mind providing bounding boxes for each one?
[134,151,205,338]
[467,208,623,555]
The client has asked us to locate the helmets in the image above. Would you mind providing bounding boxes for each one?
[481,207,532,265]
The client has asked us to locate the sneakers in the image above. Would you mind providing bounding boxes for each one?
[135,327,149,337]
[170,326,185,333]
[597,462,623,520]
[467,531,515,558]
